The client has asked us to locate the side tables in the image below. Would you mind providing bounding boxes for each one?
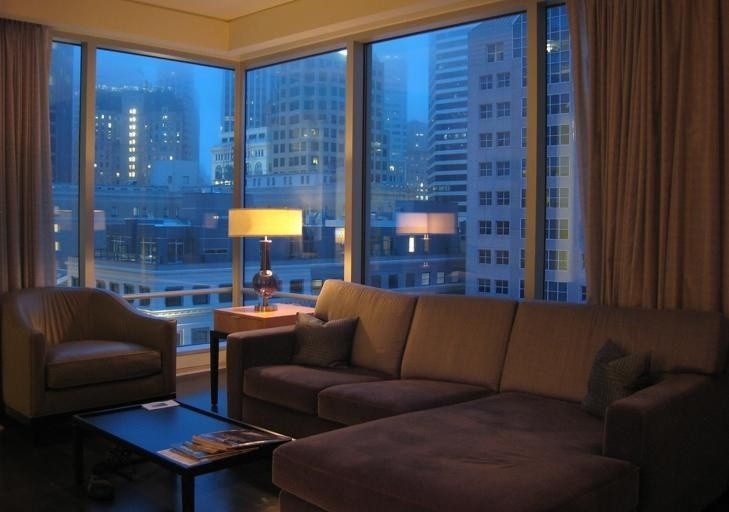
[209,303,315,413]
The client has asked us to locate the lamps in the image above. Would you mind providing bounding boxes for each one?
[228,208,303,312]
[395,213,458,269]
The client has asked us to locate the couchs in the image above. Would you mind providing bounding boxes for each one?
[227,278,729,512]
[0,287,177,430]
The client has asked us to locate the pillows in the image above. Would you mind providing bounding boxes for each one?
[294,312,360,369]
[582,339,651,420]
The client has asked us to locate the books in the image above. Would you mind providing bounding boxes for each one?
[157,429,292,469]
